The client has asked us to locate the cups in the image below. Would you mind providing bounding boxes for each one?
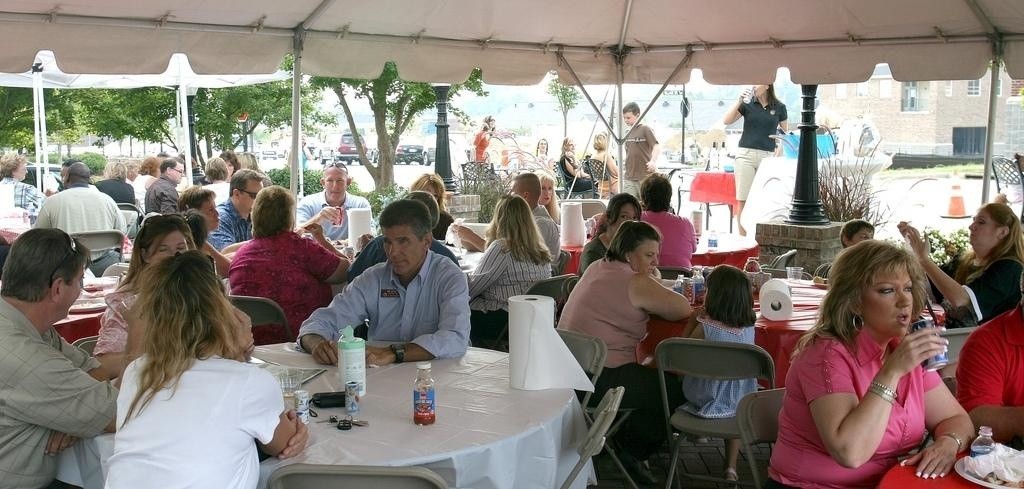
[786,266,805,294]
[344,380,361,417]
[274,366,304,400]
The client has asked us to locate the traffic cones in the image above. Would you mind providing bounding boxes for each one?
[939,169,973,218]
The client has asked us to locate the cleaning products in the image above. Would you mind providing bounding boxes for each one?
[710,140,724,171]
[948,184,966,217]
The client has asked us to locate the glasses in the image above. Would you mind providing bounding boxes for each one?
[239,188,257,200]
[49,228,77,287]
[142,213,180,229]
[615,214,638,222]
[323,162,348,171]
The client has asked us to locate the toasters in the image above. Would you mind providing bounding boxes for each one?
[709,141,718,171]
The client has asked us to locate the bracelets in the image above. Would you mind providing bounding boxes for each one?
[939,429,962,452]
[867,379,897,404]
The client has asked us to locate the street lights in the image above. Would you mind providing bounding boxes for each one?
[429,80,459,194]
[186,85,207,179]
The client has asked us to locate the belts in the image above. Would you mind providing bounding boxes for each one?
[90,258,100,264]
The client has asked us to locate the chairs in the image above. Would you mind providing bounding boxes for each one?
[677,172,736,233]
[101,263,130,278]
[559,199,607,220]
[71,336,99,358]
[551,251,571,276]
[70,230,125,262]
[478,273,579,348]
[118,203,144,239]
[737,388,785,487]
[940,327,978,365]
[266,464,446,487]
[557,328,645,489]
[655,338,776,488]
[560,276,579,303]
[658,266,690,280]
[224,295,296,343]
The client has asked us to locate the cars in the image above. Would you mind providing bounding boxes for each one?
[227,132,437,170]
[0,155,96,197]
[650,128,738,166]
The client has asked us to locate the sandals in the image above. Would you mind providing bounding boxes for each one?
[723,468,739,489]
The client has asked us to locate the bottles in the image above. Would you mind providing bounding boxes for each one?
[691,268,706,305]
[718,140,729,173]
[675,274,685,294]
[412,360,437,426]
[294,389,309,426]
[968,423,997,460]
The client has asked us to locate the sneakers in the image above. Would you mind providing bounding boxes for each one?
[614,444,659,488]
[687,434,710,444]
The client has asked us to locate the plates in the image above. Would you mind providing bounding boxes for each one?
[953,455,1024,488]
[82,277,119,291]
[66,297,108,313]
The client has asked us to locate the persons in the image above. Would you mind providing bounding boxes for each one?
[763,239,975,489]
[899,204,1024,328]
[953,271,1024,446]
[680,265,757,482]
[812,221,874,278]
[724,81,788,235]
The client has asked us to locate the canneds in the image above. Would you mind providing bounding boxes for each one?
[301,232,313,240]
[911,318,950,371]
[332,206,343,227]
[681,277,696,305]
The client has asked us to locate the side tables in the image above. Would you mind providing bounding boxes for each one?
[53,278,118,344]
